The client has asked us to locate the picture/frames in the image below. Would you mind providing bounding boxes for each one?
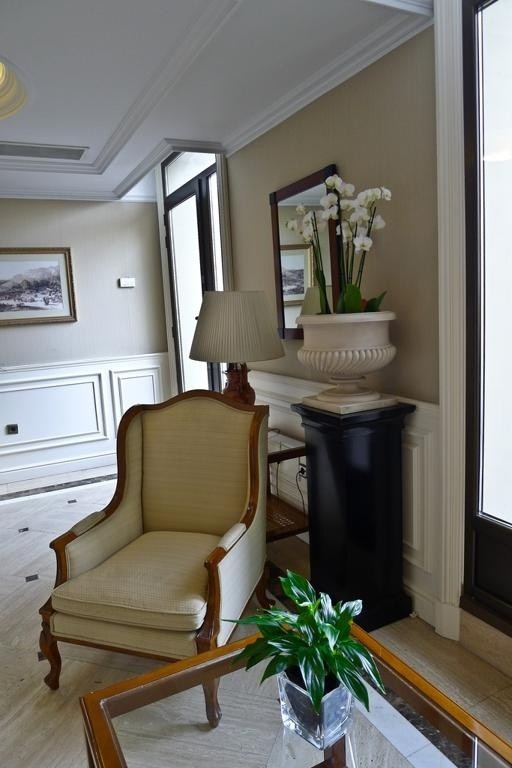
[1,246,77,328]
[280,244,315,307]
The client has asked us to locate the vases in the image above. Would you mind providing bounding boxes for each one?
[295,310,400,403]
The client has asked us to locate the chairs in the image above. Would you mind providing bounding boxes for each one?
[39,390,271,730]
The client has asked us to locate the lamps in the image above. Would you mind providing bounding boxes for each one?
[299,464,308,479]
[0,60,28,120]
[189,289,285,406]
[300,285,334,314]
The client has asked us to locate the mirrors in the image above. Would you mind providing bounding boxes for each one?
[270,164,344,339]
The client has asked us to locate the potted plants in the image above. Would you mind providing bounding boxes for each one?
[222,568,387,751]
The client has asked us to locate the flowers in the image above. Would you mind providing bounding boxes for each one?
[285,173,391,314]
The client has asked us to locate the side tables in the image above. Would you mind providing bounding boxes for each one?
[267,427,308,543]
